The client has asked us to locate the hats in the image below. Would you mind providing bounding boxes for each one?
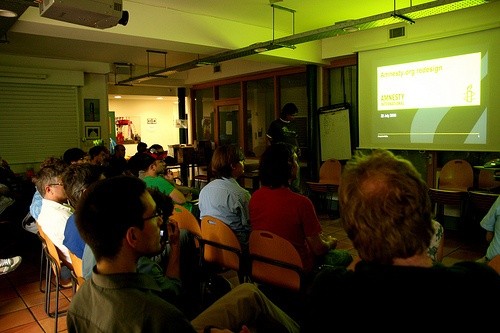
[139,151,168,171]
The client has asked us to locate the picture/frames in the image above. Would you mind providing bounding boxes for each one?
[84,99,100,122]
[85,126,101,140]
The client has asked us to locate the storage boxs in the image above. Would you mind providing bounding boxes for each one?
[168,144,195,163]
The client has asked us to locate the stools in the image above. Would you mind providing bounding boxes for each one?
[195,175,207,188]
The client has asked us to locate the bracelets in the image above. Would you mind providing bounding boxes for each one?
[203,326,213,333]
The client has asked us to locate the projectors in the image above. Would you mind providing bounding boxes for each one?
[39,0,129,29]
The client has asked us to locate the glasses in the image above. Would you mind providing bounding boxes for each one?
[141,208,164,225]
[234,158,246,165]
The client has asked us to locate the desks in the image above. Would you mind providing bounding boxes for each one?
[164,162,189,187]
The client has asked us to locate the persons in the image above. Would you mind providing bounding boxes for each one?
[480,195,500,264]
[266,103,300,155]
[198,144,251,270]
[66,177,300,333]
[300,149,500,333]
[0,143,186,313]
[248,143,354,275]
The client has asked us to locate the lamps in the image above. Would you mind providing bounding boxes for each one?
[391,0,415,25]
[270,4,296,49]
[146,50,168,78]
[195,54,214,67]
[113,62,133,86]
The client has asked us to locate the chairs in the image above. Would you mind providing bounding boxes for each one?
[169,204,306,292]
[36,222,84,333]
[306,158,500,220]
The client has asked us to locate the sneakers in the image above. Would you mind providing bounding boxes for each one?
[0,255,22,276]
